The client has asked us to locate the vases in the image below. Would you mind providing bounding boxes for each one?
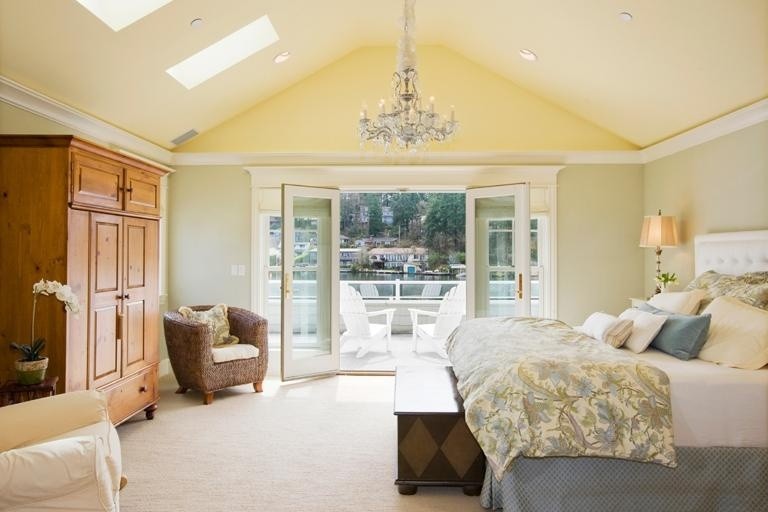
[13,357,48,385]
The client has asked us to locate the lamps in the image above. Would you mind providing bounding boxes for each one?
[639,208,680,302]
[357,0,459,154]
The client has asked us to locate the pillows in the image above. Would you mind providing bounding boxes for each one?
[580,271,767,372]
[178,304,238,347]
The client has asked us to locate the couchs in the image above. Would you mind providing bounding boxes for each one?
[0,390,125,512]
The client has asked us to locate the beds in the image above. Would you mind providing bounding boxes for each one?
[445,231,767,512]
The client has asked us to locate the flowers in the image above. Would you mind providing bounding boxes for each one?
[9,279,81,361]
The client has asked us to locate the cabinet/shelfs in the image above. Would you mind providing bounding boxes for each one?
[394,357,486,497]
[0,131,175,428]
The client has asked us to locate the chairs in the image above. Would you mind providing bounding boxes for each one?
[358,284,379,296]
[338,280,397,359]
[408,282,466,359]
[417,284,442,297]
[161,305,270,404]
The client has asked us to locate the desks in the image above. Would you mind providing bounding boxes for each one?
[360,298,444,333]
